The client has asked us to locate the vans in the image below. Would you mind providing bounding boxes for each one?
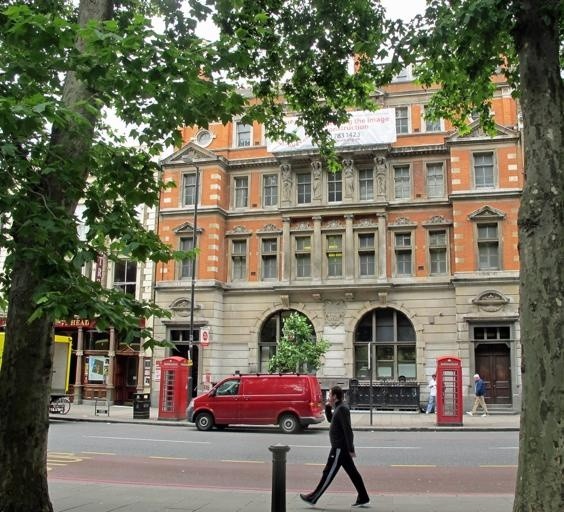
[185,370,327,432]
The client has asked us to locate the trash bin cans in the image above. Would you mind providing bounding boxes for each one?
[133,393,151,419]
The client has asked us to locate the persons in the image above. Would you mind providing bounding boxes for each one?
[298,385,372,508]
[373,156,386,196]
[342,159,355,198]
[425,374,438,415]
[465,373,491,416]
[310,161,324,200]
[278,164,292,201]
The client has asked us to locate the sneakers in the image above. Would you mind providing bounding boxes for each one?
[300,494,315,505]
[352,499,369,507]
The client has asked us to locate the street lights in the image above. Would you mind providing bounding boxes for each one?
[178,154,201,360]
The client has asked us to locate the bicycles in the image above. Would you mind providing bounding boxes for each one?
[49,395,71,416]
